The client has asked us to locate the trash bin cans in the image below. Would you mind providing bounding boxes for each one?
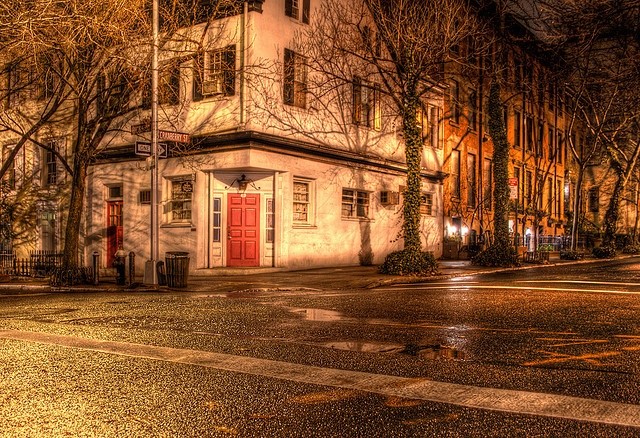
[165,251,190,288]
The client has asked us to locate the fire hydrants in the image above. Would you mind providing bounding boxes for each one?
[112,249,127,285]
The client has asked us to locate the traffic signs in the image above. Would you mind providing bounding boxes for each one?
[135,140,167,157]
[158,130,190,143]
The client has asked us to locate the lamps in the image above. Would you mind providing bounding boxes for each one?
[225,174,261,191]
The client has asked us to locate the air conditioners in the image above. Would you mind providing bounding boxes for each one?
[201,80,222,95]
